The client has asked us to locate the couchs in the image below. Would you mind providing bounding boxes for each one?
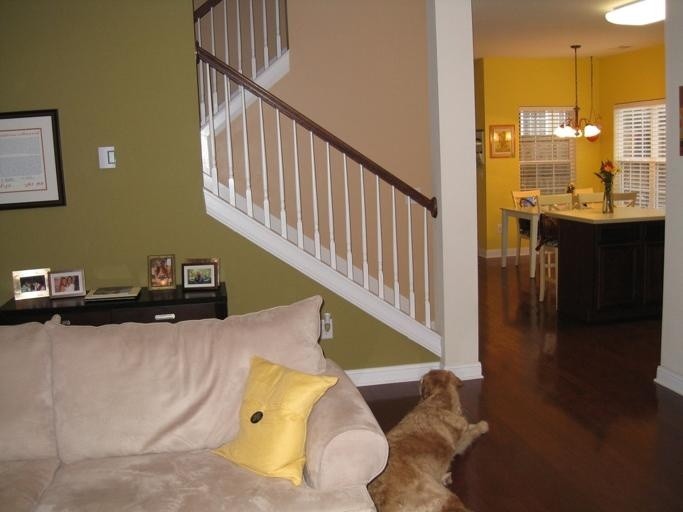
[1,296,389,511]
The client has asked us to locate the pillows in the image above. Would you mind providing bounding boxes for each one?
[210,355,339,487]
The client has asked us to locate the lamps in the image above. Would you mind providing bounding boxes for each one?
[553,45,600,138]
[604,0,666,26]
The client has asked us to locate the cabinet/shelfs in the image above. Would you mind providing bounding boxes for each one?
[545,208,665,326]
[0,282,227,326]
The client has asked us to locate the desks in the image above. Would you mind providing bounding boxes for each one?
[499,206,566,279]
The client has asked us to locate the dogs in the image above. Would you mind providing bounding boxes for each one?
[366,370,490,512]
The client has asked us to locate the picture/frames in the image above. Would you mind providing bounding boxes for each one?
[84,286,142,301]
[490,125,515,158]
[0,109,67,211]
[148,254,176,291]
[182,263,218,291]
[48,269,86,299]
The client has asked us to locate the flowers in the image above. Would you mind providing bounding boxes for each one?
[594,160,624,193]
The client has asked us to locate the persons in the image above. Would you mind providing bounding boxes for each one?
[21,280,31,292]
[193,269,204,281]
[150,260,171,287]
[32,280,45,291]
[65,276,74,291]
[58,276,67,291]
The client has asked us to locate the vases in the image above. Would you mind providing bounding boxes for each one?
[602,193,614,218]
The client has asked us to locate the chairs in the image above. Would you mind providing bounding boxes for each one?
[577,193,636,209]
[572,187,593,207]
[511,189,541,266]
[536,193,572,310]
